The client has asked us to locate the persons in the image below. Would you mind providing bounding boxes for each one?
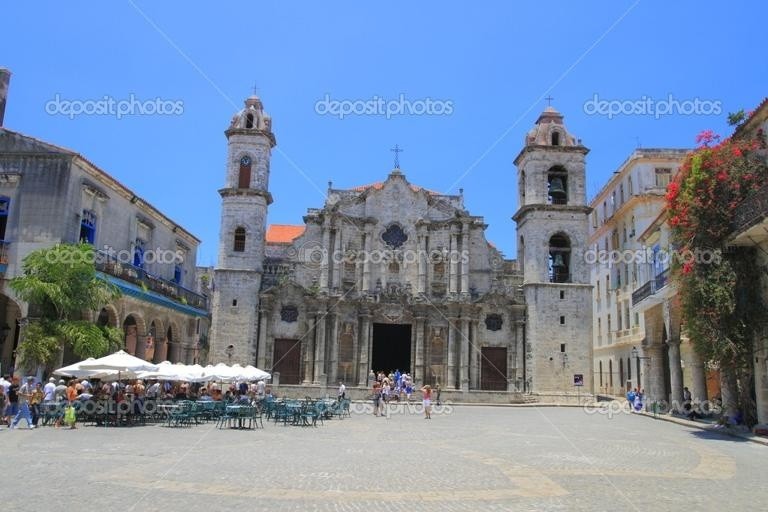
[365,368,443,420]
[337,381,346,402]
[0,374,272,431]
[619,384,757,433]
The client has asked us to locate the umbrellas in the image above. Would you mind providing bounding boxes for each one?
[51,349,272,395]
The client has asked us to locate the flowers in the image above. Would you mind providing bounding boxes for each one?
[665,128,768,275]
